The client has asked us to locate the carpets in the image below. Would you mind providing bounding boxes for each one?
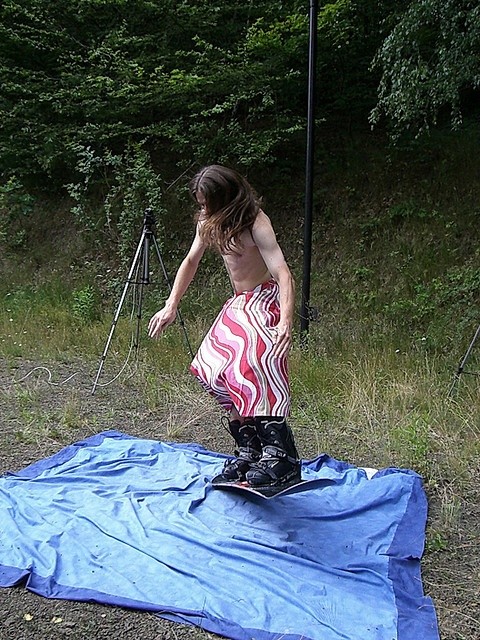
[2,427,439,635]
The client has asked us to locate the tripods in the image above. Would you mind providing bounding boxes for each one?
[89,206,195,396]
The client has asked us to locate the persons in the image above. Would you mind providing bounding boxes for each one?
[147,165,300,487]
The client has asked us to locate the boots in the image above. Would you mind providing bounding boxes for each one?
[221,416,263,480]
[246,415,301,487]
